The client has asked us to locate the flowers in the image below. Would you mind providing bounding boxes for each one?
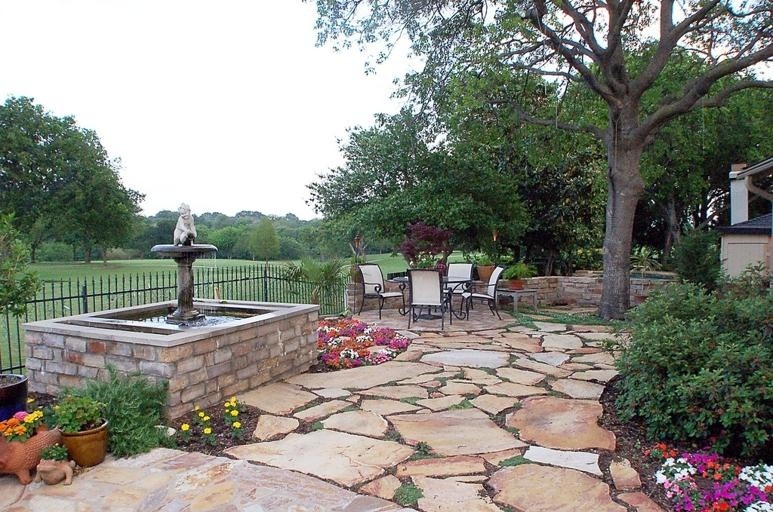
[0,407,42,441]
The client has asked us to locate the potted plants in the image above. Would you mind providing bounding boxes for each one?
[503,262,537,290]
[34,443,79,485]
[57,394,109,467]
[474,254,495,282]
[0,212,28,420]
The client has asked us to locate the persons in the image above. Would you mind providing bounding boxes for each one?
[174,203,197,247]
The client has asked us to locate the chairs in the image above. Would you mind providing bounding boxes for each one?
[357,263,505,330]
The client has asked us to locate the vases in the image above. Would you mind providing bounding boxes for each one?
[0,427,59,483]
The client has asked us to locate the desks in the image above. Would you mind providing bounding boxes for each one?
[496,288,538,314]
